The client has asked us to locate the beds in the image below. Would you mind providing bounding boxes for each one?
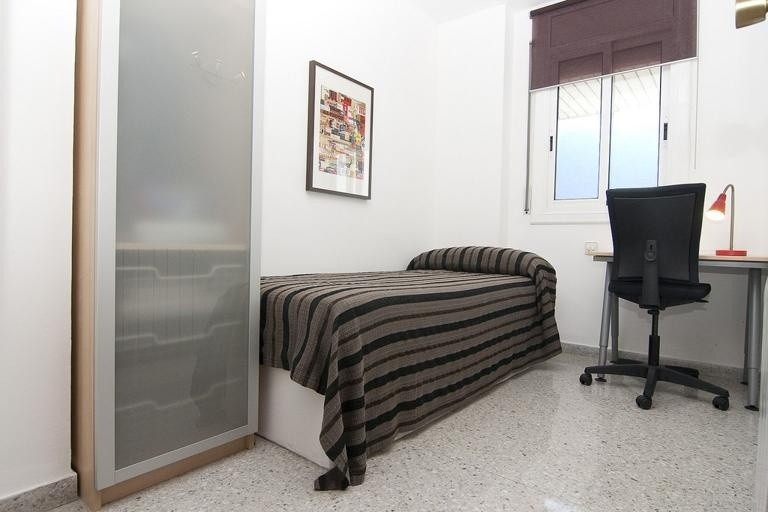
[259,271,556,469]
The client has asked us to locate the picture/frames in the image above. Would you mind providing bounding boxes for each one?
[304,58,375,202]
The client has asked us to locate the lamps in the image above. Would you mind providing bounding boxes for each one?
[706,184,748,256]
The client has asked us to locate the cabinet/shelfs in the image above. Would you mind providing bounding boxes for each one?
[75,0,263,507]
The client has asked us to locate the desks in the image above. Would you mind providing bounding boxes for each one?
[584,249,768,413]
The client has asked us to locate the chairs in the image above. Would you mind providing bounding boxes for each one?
[580,180,730,411]
